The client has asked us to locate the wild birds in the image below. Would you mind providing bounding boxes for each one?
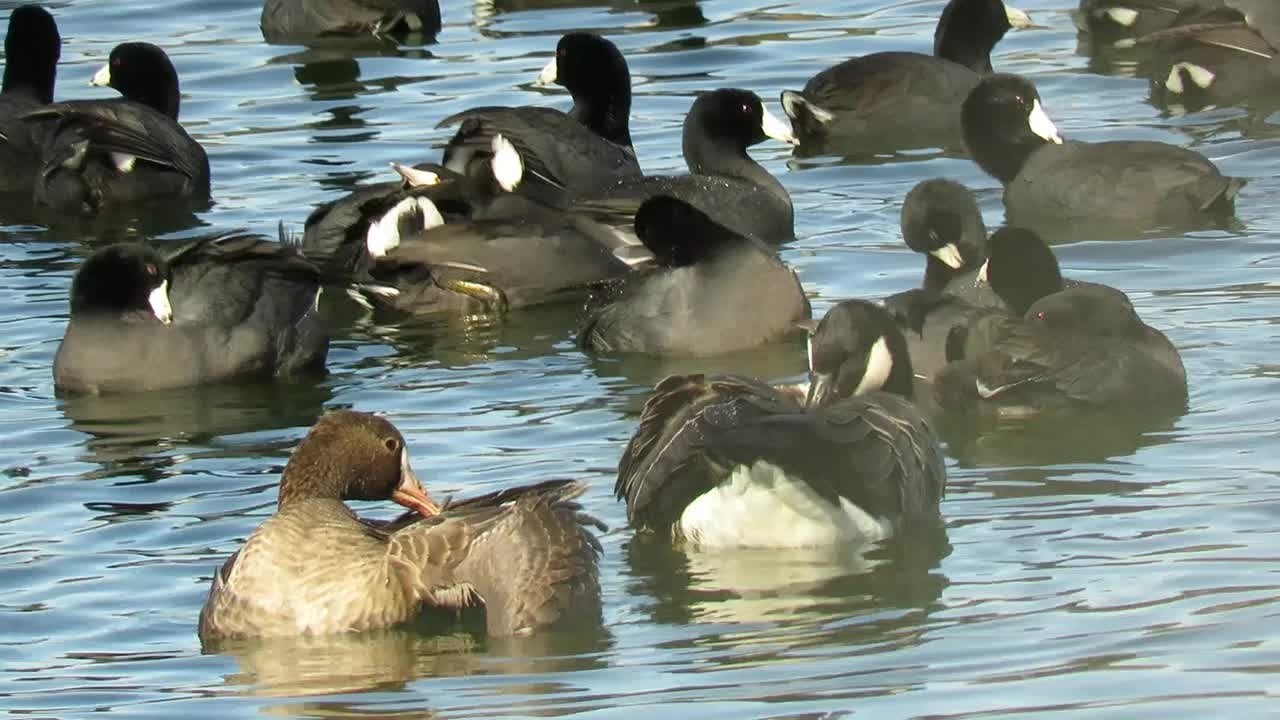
[51,218,337,398]
[0,7,212,229]
[302,31,796,329]
[561,194,813,359]
[198,410,609,657]
[612,297,946,560]
[259,0,442,43]
[780,0,1280,245]
[788,178,1188,421]
